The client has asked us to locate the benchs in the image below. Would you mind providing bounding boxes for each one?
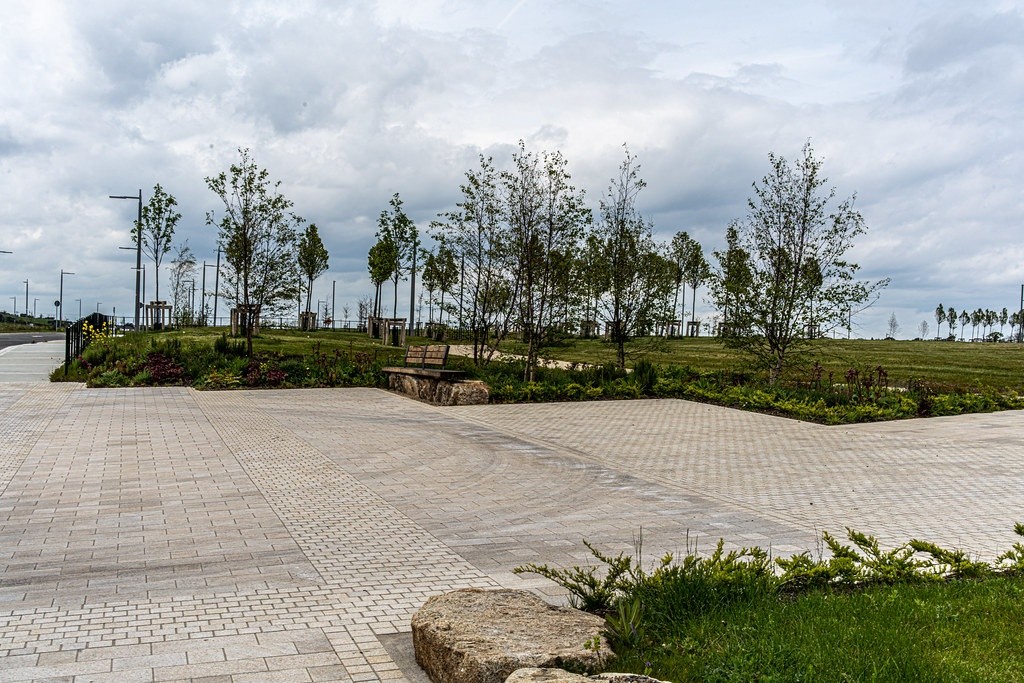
[382,344,465,378]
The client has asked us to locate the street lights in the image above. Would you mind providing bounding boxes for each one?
[59,269,76,328]
[96,302,102,325]
[109,189,143,333]
[130,264,146,331]
[183,278,195,317]
[202,260,217,326]
[23,279,28,325]
[34,298,39,318]
[10,296,17,323]
[75,298,81,319]
